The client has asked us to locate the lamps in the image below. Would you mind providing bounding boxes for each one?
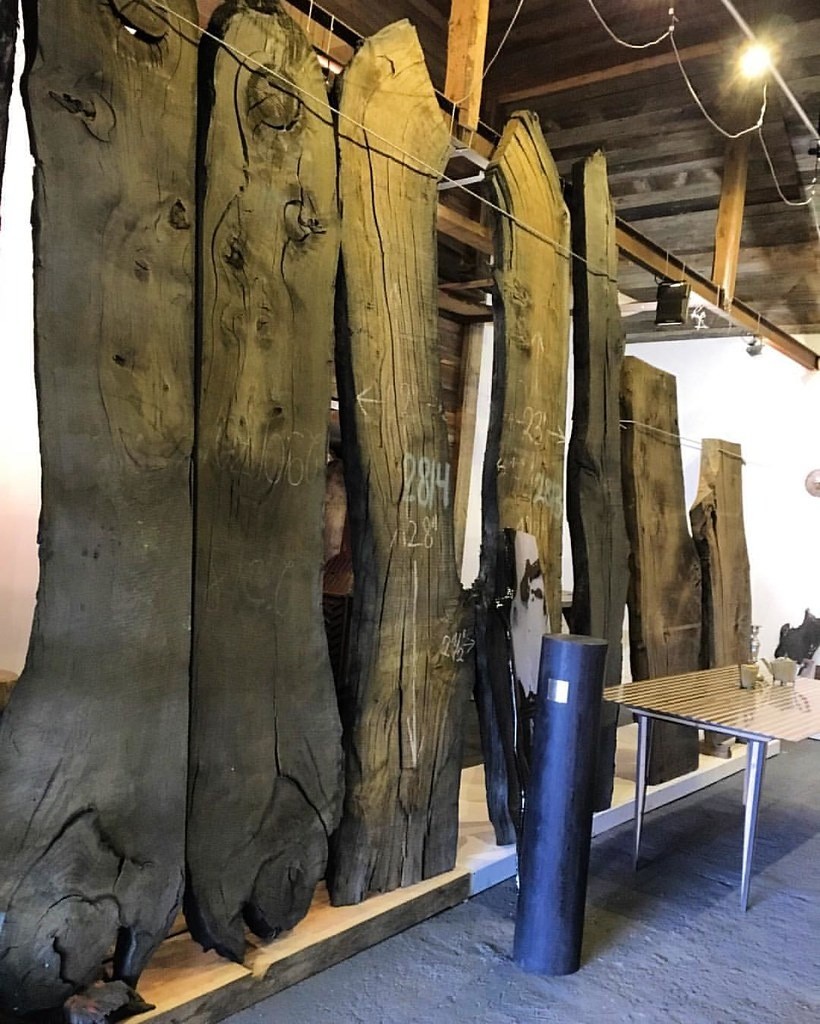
[653,279,692,327]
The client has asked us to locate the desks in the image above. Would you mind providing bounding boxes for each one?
[599,661,820,912]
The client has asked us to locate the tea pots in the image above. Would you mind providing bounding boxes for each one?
[760,653,806,684]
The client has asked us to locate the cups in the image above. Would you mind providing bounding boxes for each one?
[740,663,759,690]
